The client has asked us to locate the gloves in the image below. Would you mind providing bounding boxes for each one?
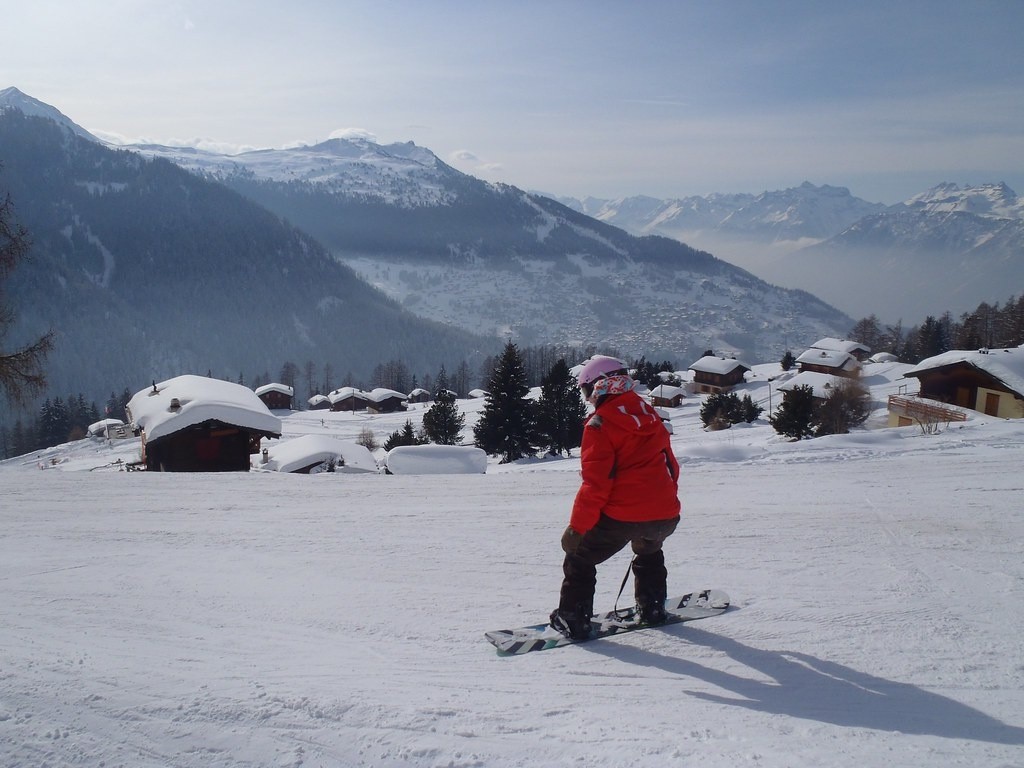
[561,525,584,556]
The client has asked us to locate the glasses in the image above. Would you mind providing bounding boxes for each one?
[581,385,594,397]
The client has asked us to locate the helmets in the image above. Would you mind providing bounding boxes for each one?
[578,356,630,388]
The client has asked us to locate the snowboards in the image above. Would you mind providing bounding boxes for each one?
[485,587,730,658]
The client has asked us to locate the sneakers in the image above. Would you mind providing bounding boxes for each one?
[635,601,667,625]
[549,608,593,639]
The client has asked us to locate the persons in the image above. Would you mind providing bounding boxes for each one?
[550,357,681,640]
